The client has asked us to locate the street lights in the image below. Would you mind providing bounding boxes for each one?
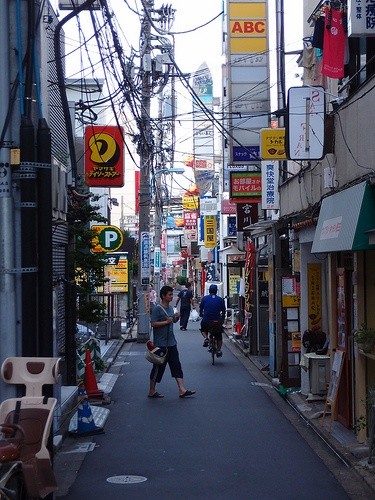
[137,168,185,342]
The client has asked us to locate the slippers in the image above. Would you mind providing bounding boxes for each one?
[178,390,196,398]
[148,392,165,398]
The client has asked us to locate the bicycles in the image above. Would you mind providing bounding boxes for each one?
[200,314,226,365]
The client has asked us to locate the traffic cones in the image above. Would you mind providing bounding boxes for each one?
[83,348,103,397]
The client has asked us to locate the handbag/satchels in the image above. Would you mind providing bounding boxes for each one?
[145,341,168,364]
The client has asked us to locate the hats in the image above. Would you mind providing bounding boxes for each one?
[207,284,219,290]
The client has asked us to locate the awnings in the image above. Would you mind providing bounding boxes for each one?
[310,181,371,253]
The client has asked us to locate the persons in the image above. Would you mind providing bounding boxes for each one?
[149,284,157,316]
[200,284,226,358]
[147,286,196,398]
[174,283,196,331]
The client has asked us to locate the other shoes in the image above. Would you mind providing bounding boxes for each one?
[215,351,223,358]
[203,338,210,348]
